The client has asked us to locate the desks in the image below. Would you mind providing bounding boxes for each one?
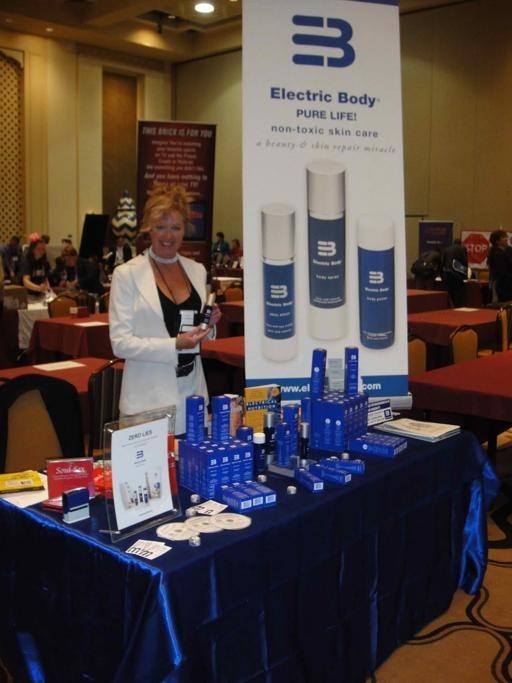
[2,247,244,350]
[409,349,510,503]
[1,415,501,682]
[31,313,117,358]
[200,336,245,393]
[2,356,123,414]
[408,306,504,365]
[463,279,494,305]
[407,288,450,312]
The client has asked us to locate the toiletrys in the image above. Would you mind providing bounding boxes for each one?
[253,432,268,478]
[264,411,276,463]
[132,484,148,505]
[298,422,311,465]
[358,218,397,349]
[307,159,348,340]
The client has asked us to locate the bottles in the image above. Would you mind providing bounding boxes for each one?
[299,422,313,469]
[302,152,349,345]
[262,410,277,463]
[354,210,398,350]
[251,431,265,465]
[132,484,149,506]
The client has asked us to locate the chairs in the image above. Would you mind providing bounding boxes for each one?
[2,373,86,477]
[448,323,478,366]
[408,335,426,378]
[86,359,127,464]
[224,279,243,301]
[48,294,79,319]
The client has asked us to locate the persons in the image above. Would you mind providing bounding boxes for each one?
[1,230,243,302]
[485,230,511,301]
[109,182,222,437]
[440,239,468,307]
[410,247,440,291]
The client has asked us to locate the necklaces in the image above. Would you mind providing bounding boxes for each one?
[149,245,179,263]
